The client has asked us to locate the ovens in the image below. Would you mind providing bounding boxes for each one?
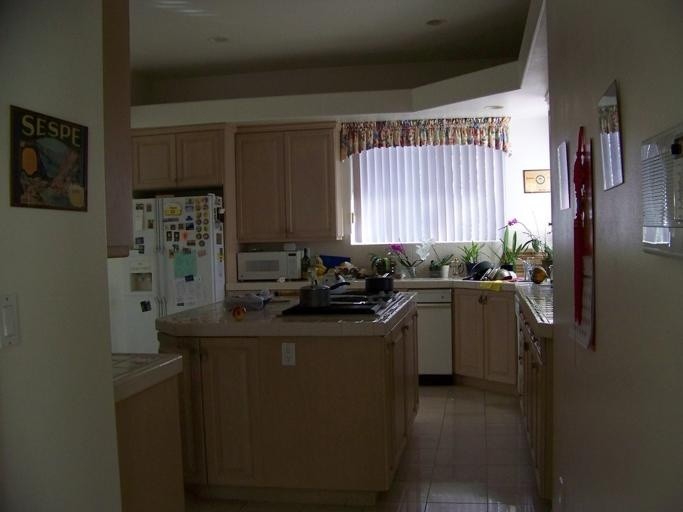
[408,289,452,376]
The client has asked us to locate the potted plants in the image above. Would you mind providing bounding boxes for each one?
[429,226,551,279]
[368,252,397,275]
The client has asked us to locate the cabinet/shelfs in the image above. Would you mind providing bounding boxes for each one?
[235,129,343,243]
[511,297,552,497]
[157,334,258,489]
[132,130,233,189]
[259,308,419,491]
[453,288,518,384]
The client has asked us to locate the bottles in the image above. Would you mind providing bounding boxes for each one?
[301,247,312,280]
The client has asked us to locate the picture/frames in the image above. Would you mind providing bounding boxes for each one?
[7,104,89,214]
[522,169,550,194]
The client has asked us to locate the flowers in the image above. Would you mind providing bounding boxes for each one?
[388,237,438,268]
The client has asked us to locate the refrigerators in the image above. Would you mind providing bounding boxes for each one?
[107,192,225,356]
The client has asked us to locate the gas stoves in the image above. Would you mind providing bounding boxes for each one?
[280,290,400,314]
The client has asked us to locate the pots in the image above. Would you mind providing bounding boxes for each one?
[462,261,517,281]
[364,275,392,295]
[298,279,351,310]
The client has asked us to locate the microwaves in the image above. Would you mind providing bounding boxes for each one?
[235,249,312,281]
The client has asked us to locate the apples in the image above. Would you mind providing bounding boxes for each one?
[233,305,247,320]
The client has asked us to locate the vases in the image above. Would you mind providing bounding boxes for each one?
[399,267,416,279]
